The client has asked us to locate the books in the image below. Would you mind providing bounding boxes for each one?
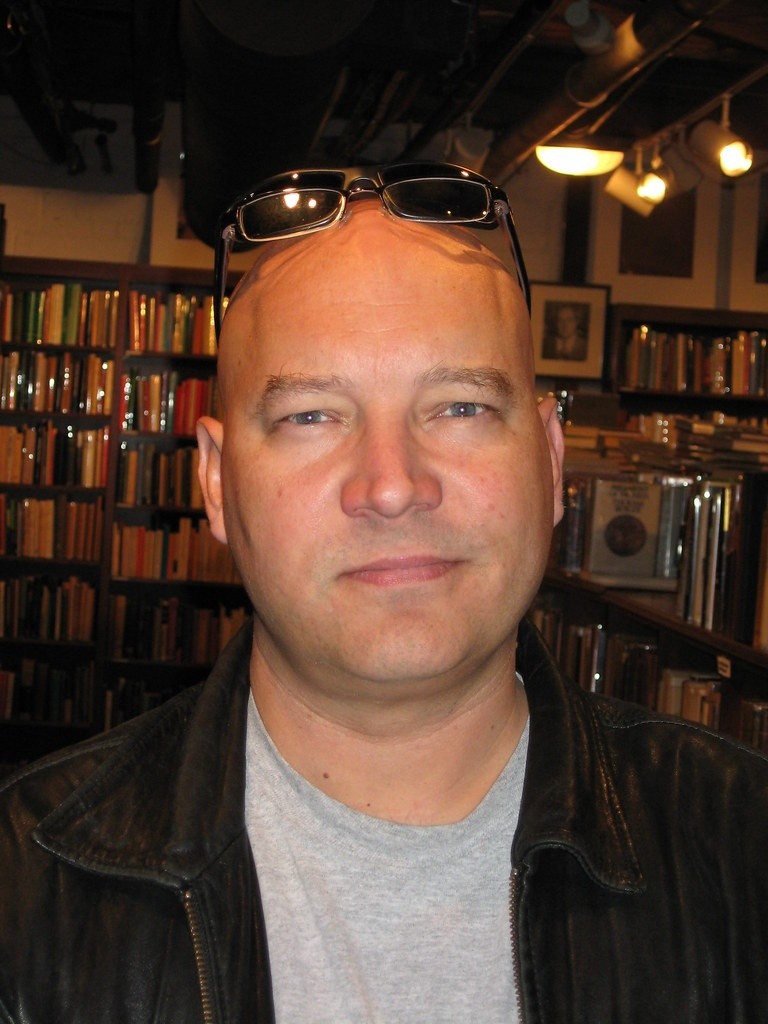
[0,280,768,757]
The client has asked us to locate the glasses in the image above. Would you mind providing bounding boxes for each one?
[214,159,532,348]
[221,178,524,296]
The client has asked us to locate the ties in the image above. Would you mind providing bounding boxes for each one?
[560,341,567,354]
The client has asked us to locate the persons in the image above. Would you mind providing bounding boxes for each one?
[552,301,587,356]
[0,154,768,1024]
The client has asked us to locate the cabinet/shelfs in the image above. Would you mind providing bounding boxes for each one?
[535,302,768,757]
[1,255,251,739]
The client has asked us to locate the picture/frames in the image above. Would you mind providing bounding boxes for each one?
[528,280,611,384]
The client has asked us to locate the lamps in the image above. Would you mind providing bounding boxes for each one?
[690,94,753,177]
[443,113,491,173]
[604,141,674,218]
